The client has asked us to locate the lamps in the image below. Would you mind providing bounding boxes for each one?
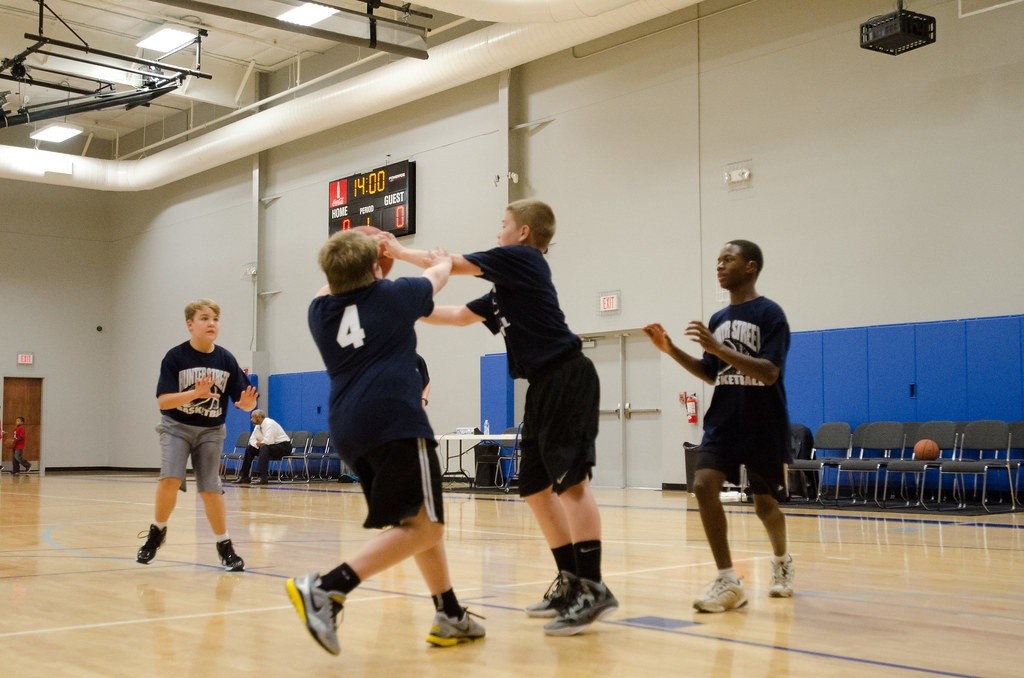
[137,21,199,52]
[276,3,340,27]
[724,165,751,183]
[30,121,84,143]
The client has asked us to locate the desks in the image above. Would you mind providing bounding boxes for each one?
[434,434,524,494]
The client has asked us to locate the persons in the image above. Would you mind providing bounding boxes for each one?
[12,417,31,475]
[287,229,485,654]
[136,297,260,571]
[372,200,619,636]
[233,409,292,485]
[643,240,795,613]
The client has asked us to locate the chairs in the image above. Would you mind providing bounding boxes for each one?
[474,425,523,487]
[221,430,346,484]
[743,420,1024,514]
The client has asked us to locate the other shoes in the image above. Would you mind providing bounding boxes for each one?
[0,466,4,470]
[251,477,268,485]
[230,476,251,484]
[11,470,20,475]
[25,463,32,472]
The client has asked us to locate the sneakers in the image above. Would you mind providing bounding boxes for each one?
[135,524,167,565]
[524,569,579,617]
[769,553,795,598]
[426,606,486,647]
[216,538,245,570]
[543,578,619,637]
[692,577,748,612]
[285,572,347,656]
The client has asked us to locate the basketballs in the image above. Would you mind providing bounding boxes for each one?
[353,225,394,277]
[5,438,15,449]
